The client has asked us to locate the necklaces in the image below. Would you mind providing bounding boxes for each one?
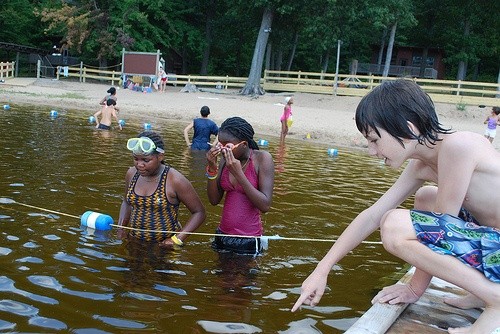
[241,156,250,169]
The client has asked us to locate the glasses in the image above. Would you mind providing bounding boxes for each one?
[216,141,246,153]
[127,136,165,154]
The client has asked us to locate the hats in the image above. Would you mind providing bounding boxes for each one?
[285,97,291,103]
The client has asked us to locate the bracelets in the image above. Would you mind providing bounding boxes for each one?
[206,166,218,180]
[171,234,183,245]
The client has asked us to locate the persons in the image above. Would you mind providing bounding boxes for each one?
[483,106,500,144]
[94,99,123,131]
[290,78,500,334]
[100,87,119,116]
[117,132,206,246]
[159,66,168,93]
[280,97,293,141]
[184,106,218,149]
[205,116,273,261]
